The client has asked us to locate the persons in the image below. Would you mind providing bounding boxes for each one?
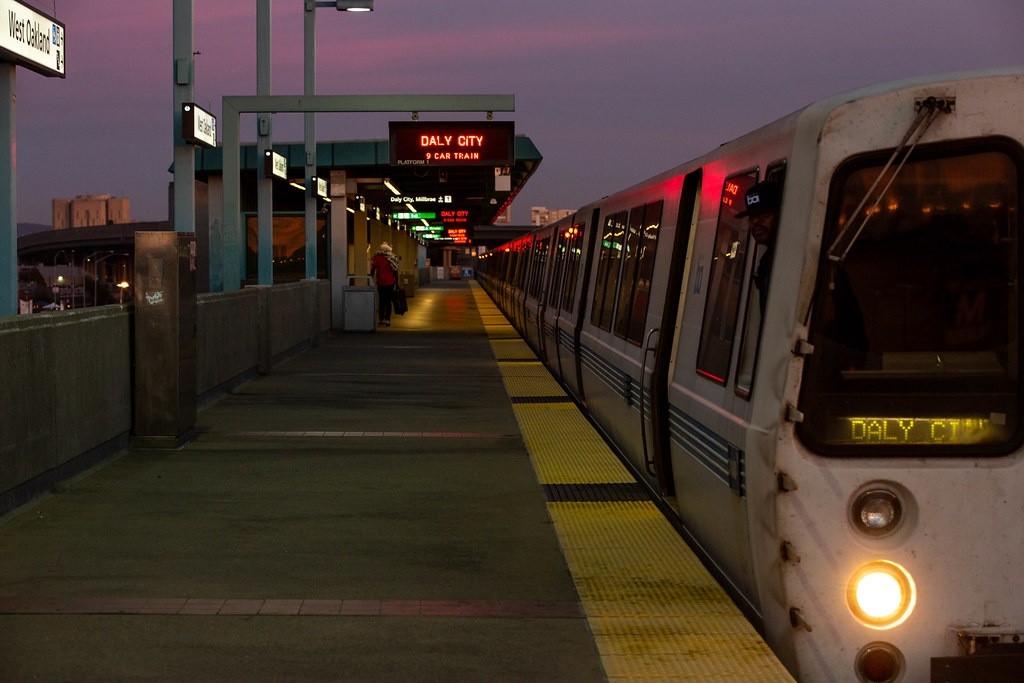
[371,243,400,326]
[735,183,867,372]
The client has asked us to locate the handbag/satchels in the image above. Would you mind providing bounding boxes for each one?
[392,285,408,315]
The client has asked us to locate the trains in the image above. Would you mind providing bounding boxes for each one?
[474,66,1023,683]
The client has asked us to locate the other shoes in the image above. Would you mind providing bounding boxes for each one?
[377,320,386,324]
[386,320,391,326]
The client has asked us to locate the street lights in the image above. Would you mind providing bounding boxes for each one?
[83,249,115,309]
[95,253,131,307]
[53,250,65,304]
[303,0,375,280]
